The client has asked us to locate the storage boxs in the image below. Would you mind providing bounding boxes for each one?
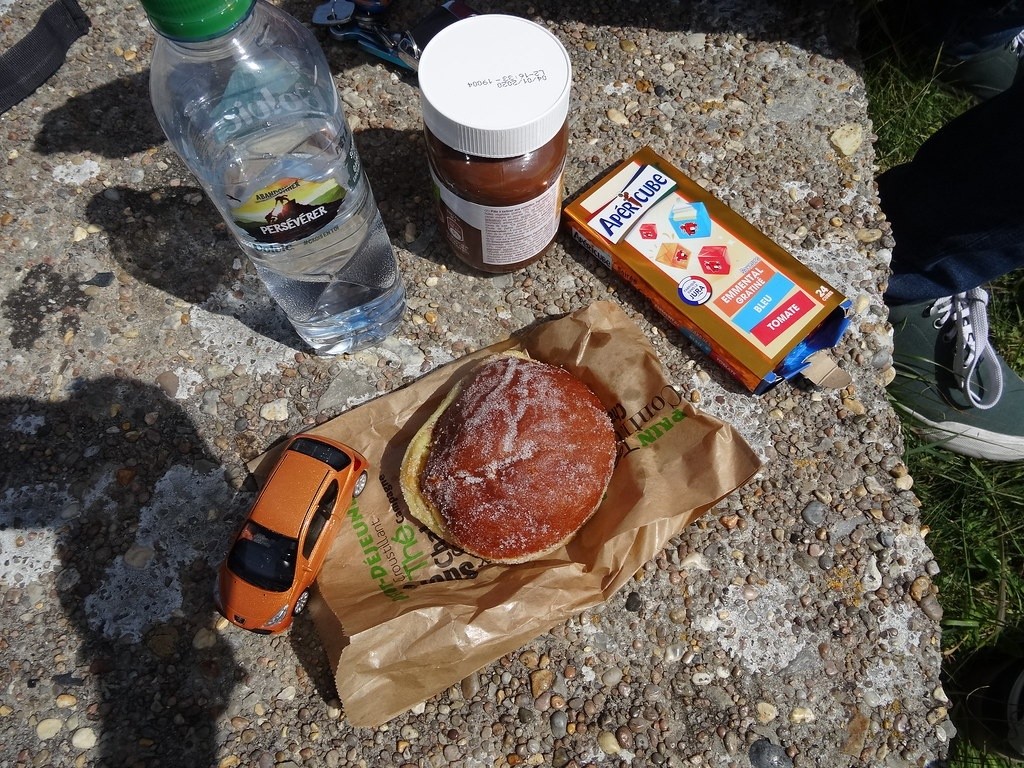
[567,145,847,390]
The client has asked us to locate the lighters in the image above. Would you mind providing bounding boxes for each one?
[413,0,479,50]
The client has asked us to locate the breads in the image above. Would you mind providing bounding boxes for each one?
[398,349,617,564]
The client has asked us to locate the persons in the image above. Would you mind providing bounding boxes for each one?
[861,0,1024,462]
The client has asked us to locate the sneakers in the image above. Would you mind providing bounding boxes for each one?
[888,284,1023,462]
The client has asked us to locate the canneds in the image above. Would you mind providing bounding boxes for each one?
[415,13,570,274]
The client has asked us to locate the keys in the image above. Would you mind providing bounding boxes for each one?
[312,0,422,73]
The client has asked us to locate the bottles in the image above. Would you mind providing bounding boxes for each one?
[418,14,571,274]
[140,0,406,354]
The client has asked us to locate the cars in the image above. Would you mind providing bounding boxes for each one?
[210,433,370,635]
